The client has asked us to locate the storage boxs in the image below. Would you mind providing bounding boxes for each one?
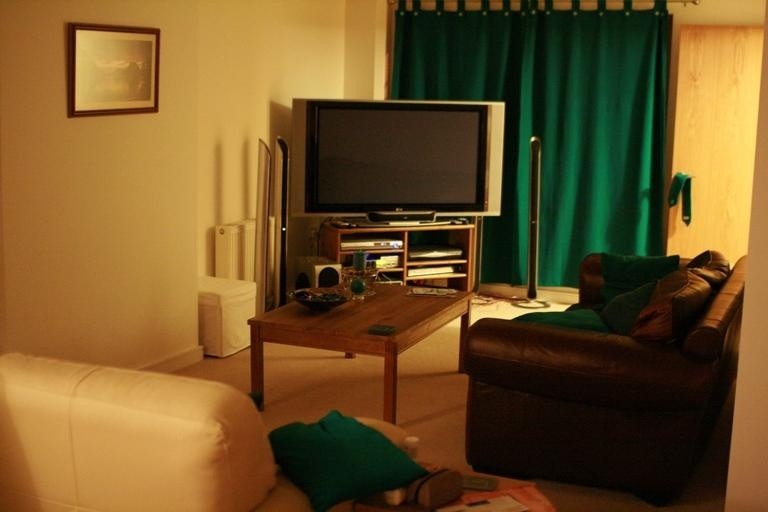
[199,276,259,357]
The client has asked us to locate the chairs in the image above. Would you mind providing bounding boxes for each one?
[462,251,749,506]
[0,348,418,510]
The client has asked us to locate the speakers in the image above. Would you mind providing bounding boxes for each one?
[294,255,342,289]
[472,215,484,294]
[528,135,542,299]
[255,138,272,315]
[274,136,288,311]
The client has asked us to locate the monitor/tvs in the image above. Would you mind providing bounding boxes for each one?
[289,98,507,229]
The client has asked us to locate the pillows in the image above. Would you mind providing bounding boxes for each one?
[268,409,427,511]
[508,254,681,332]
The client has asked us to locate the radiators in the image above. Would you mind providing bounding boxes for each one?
[214,216,281,318]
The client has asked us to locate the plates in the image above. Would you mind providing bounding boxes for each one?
[287,286,351,310]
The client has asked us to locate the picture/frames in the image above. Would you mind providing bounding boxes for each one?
[66,22,161,117]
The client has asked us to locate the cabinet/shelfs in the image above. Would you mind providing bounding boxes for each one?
[318,221,476,297]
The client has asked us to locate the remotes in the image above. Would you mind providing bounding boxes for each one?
[329,220,349,228]
